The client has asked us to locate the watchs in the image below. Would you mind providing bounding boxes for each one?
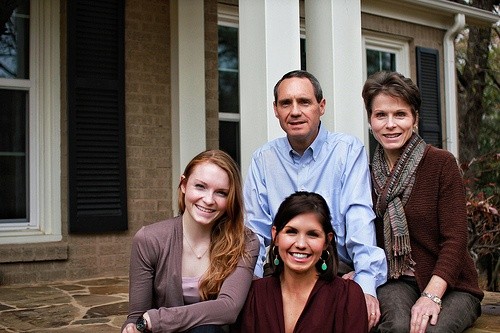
[136,311,152,333]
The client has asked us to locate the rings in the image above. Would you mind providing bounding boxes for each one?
[371,312,376,315]
[422,314,430,317]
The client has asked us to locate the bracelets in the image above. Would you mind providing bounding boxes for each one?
[420,292,442,305]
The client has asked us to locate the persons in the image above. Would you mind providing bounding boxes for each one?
[121,150,261,333]
[342,72,484,333]
[241,70,388,330]
[234,191,369,333]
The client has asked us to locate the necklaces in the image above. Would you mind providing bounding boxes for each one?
[183,234,209,259]
